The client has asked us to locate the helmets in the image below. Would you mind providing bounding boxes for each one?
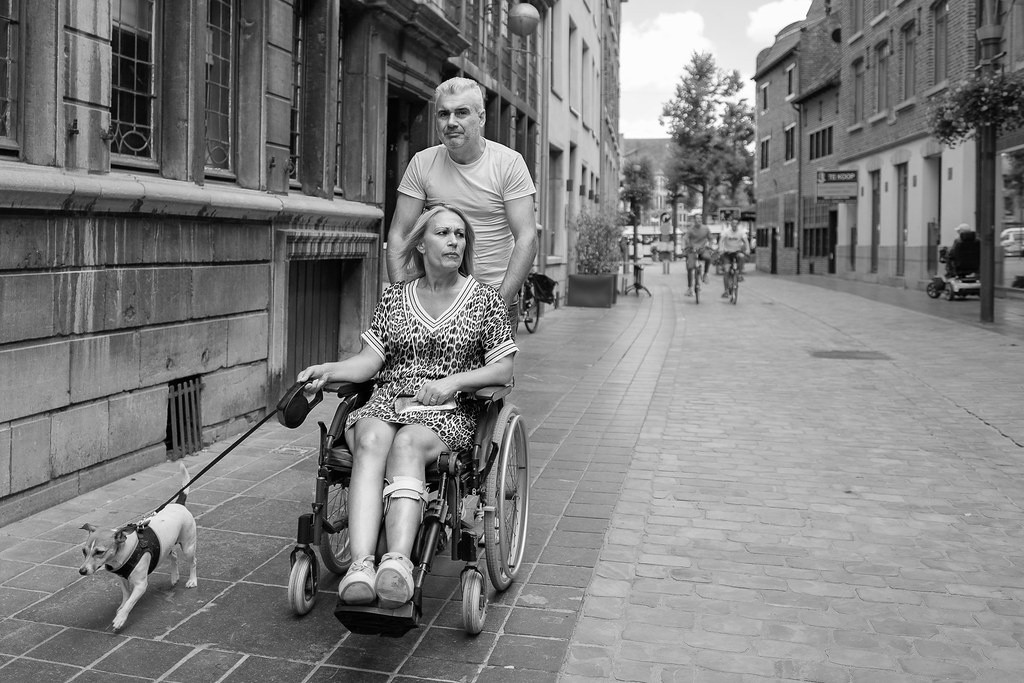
[955,223,971,233]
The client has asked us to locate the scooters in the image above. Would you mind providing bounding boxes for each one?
[926,245,982,301]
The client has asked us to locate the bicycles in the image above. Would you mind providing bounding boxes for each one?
[724,250,743,304]
[515,275,539,333]
[684,246,713,304]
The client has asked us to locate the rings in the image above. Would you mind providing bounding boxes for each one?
[430,398,437,404]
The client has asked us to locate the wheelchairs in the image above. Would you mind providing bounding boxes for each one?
[287,378,531,635]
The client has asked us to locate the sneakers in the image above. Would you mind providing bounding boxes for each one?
[374,552,414,610]
[338,555,376,605]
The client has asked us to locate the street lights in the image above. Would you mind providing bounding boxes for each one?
[632,162,641,262]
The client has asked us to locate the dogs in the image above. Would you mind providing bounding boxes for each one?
[79,461,197,633]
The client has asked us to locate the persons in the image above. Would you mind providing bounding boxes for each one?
[718,219,750,298]
[945,223,971,277]
[297,205,520,603]
[684,213,714,298]
[386,77,538,546]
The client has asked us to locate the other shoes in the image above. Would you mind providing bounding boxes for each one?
[737,276,743,282]
[704,273,709,284]
[685,289,692,295]
[722,293,729,298]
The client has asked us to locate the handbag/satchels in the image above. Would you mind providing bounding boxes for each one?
[526,272,558,304]
[518,283,532,301]
